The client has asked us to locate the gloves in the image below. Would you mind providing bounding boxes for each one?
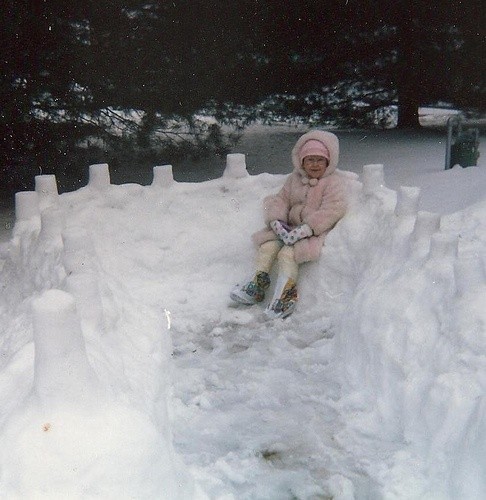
[270,220,292,241]
[283,224,313,245]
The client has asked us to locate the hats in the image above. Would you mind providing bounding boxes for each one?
[300,140,329,164]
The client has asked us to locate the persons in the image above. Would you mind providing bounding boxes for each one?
[230,130,350,319]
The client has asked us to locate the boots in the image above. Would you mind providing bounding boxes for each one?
[230,270,271,304]
[264,276,298,321]
